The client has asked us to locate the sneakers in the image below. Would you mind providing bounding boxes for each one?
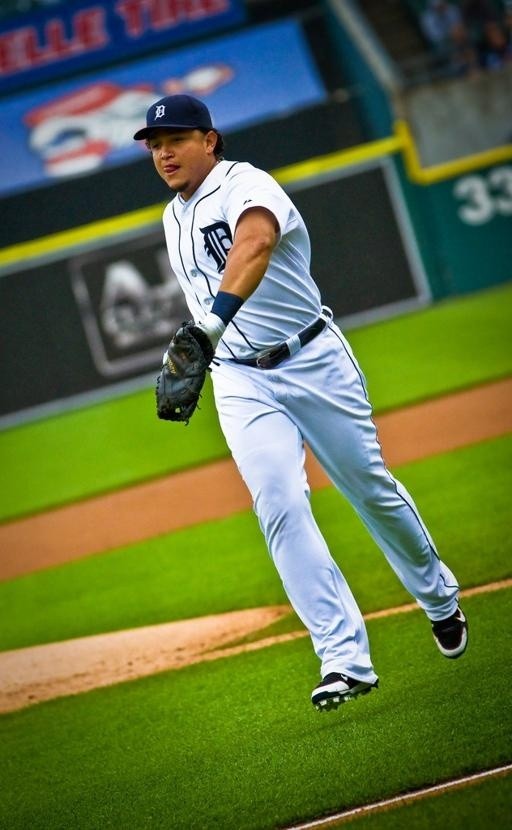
[310,666,376,713]
[431,605,468,659]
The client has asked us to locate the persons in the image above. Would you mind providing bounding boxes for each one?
[413,0,512,80]
[130,92,474,711]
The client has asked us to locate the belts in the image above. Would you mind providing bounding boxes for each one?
[225,309,333,371]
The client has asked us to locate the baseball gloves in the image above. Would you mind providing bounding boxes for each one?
[156,320,214,425]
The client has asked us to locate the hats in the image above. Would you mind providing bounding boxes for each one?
[133,94,214,140]
